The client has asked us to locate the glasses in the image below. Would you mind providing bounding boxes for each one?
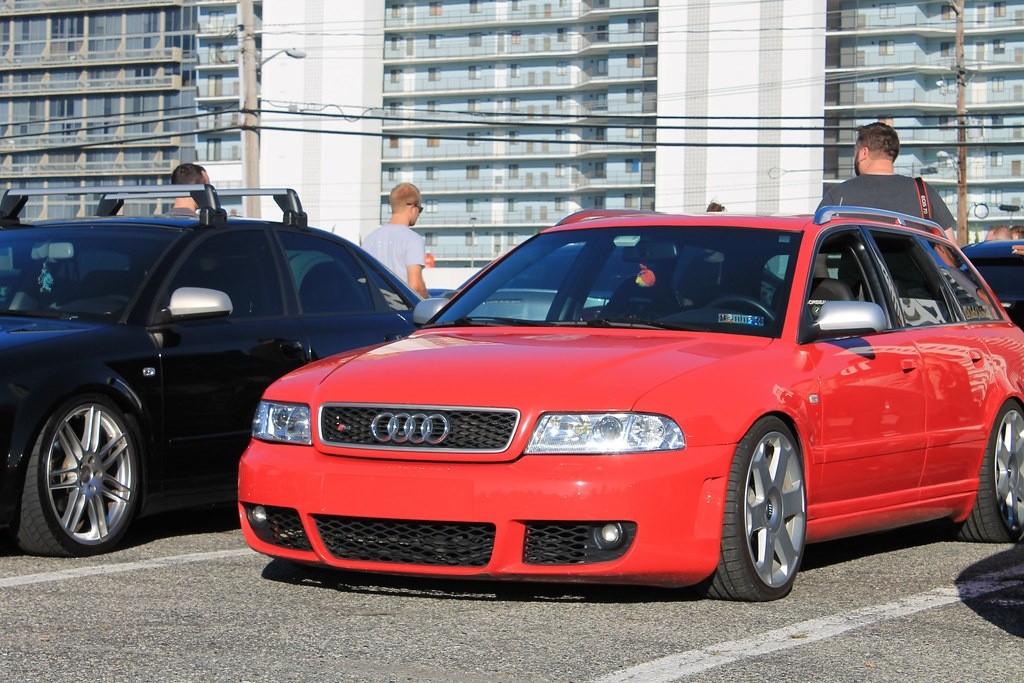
[407,204,424,213]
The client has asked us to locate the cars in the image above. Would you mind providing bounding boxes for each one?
[440,234,641,320]
[238,204,1024,604]
[961,239,1024,333]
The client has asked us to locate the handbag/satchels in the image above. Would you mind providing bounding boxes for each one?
[915,177,959,269]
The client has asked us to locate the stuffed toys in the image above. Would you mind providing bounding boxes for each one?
[635,264,655,287]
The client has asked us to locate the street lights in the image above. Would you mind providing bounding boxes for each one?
[241,46,307,217]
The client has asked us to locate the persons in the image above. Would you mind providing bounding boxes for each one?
[360,182,429,310]
[161,163,211,219]
[927,239,992,308]
[815,122,958,278]
[985,225,1024,240]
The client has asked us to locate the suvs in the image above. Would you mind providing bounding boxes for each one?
[0,183,421,559]
